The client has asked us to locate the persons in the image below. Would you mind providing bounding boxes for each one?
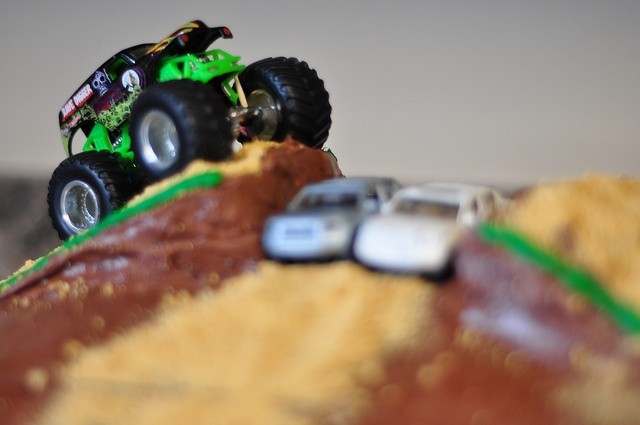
[354,181,507,275]
[45,18,331,241]
[261,174,404,266]
